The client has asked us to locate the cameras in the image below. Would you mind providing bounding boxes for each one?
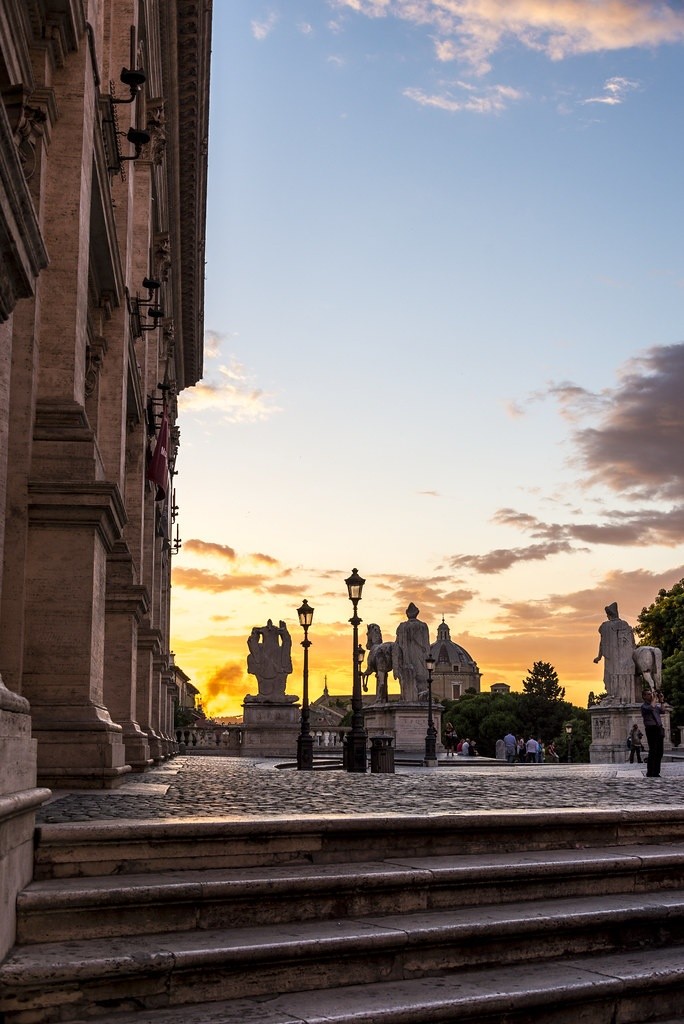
[655,689,661,694]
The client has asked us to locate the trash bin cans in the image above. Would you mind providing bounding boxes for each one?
[369,734,396,774]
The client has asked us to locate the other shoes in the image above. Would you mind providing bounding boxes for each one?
[646,771,662,778]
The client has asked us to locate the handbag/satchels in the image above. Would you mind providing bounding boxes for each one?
[659,726,665,738]
[627,736,632,748]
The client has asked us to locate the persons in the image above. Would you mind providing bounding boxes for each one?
[593,602,637,704]
[442,723,479,758]
[640,688,665,778]
[395,602,431,702]
[504,730,556,764]
[247,619,293,673]
[629,724,643,764]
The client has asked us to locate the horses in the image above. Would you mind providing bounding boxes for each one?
[363,624,403,703]
[634,646,662,703]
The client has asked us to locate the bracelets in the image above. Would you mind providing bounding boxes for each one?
[661,701,664,704]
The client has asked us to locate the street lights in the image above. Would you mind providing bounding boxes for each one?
[344,568,370,773]
[295,598,316,770]
[424,652,439,761]
[565,722,572,762]
